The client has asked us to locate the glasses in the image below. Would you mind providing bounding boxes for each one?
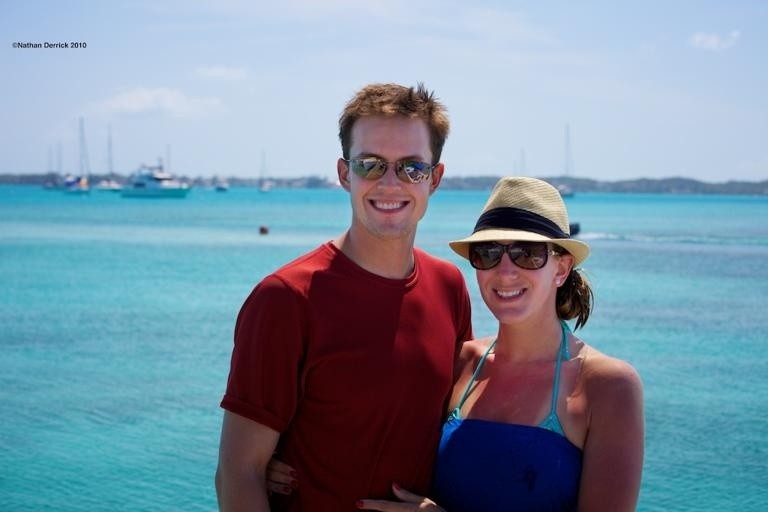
[344,154,439,185]
[469,241,561,270]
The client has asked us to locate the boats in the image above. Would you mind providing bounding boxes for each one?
[214,181,228,192]
[44,177,61,191]
[119,165,190,200]
[556,184,574,200]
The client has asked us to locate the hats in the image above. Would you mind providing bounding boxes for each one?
[449,177,591,270]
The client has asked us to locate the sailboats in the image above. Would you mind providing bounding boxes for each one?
[96,124,122,191]
[257,152,273,194]
[63,117,92,194]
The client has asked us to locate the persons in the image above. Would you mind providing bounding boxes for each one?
[215,81,475,512]
[354,176,644,512]
